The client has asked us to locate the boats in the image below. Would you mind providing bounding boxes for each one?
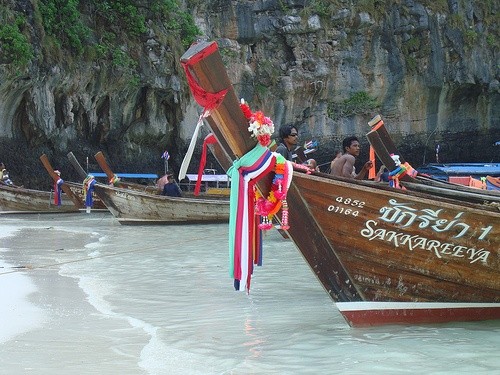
[365,130,499,204]
[182,41,500,328]
[365,115,499,204]
[0,151,231,227]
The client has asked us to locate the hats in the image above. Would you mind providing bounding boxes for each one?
[167,176,174,179]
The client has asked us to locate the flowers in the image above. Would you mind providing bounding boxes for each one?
[240,102,275,146]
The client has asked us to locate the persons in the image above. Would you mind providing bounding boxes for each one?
[2,169,18,187]
[332,136,374,183]
[0,162,24,188]
[276,124,320,174]
[163,176,182,198]
[158,172,184,194]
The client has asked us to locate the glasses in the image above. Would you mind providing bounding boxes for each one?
[289,133,298,137]
